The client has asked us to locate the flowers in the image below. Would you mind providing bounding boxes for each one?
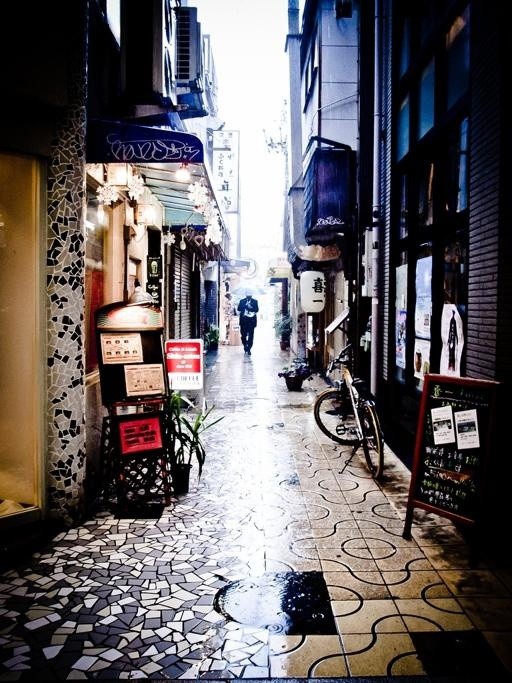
[276,354,313,376]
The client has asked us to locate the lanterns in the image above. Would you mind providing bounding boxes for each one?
[300,268,326,312]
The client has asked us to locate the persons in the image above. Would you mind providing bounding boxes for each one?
[222,291,235,345]
[236,288,257,356]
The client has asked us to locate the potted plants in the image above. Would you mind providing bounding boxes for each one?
[272,316,292,350]
[167,393,225,493]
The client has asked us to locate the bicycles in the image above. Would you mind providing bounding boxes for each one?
[312,341,385,482]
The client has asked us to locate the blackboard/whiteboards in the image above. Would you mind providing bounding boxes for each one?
[411,374,500,524]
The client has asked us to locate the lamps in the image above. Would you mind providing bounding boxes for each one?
[175,163,191,181]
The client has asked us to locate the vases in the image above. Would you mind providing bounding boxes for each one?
[283,377,307,389]
